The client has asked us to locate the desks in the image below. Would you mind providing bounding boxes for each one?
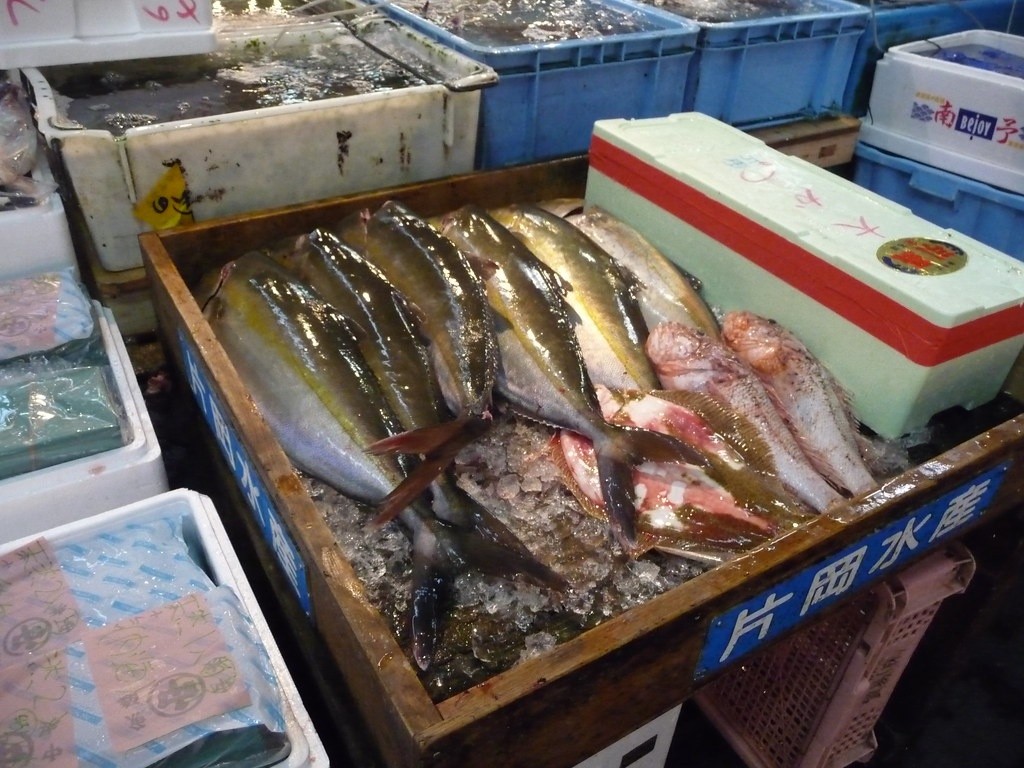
[105,118,861,374]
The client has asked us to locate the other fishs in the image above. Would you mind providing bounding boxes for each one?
[190,193,890,676]
[0,77,46,212]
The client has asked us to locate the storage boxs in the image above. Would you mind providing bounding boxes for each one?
[858,27,1024,264]
[0,486,331,768]
[651,0,873,133]
[584,111,1024,448]
[0,300,169,541]
[373,1,701,171]
[134,152,1023,768]
[22,13,501,278]
[844,0,1024,116]
[0,158,82,282]
